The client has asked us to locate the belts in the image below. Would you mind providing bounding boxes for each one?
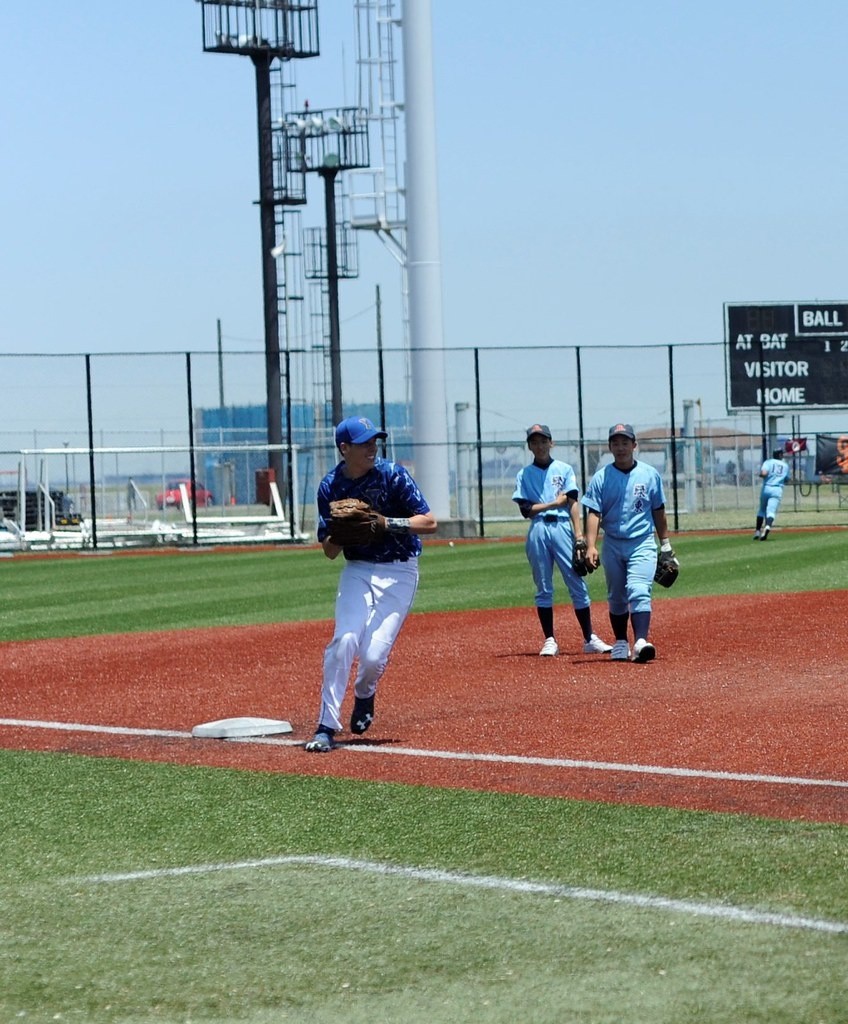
[360,556,410,563]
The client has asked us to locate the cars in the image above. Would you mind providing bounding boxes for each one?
[154,479,214,510]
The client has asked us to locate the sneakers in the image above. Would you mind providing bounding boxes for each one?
[305,725,337,752]
[349,694,375,734]
[631,638,656,663]
[583,634,613,654]
[610,640,631,659]
[540,636,559,656]
[760,525,771,541]
[753,530,760,540]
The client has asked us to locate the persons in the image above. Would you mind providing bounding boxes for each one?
[512,424,614,656]
[754,461,763,485]
[581,423,678,662]
[753,447,791,541]
[305,417,439,753]
[726,461,737,486]
[79,483,87,512]
[126,477,137,511]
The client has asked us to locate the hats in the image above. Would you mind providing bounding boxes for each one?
[608,423,635,442]
[335,415,388,447]
[773,447,786,458]
[526,423,552,442]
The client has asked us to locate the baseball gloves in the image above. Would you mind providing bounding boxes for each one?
[653,548,680,589]
[571,539,601,577]
[328,498,386,537]
